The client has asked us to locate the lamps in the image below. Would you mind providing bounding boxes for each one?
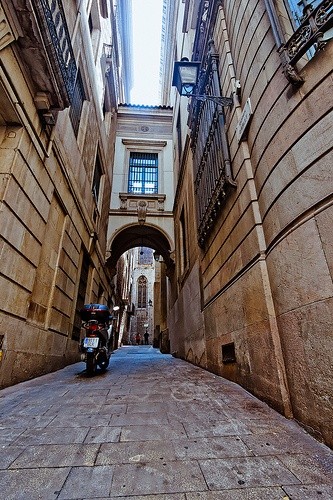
[172,57,233,107]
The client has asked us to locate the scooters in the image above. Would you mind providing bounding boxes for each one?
[78,304,120,377]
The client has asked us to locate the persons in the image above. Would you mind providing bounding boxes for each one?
[135,329,149,345]
